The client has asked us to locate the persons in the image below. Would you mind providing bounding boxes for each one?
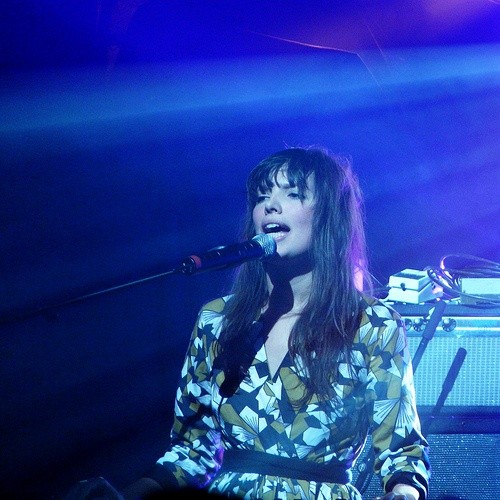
[57,146,435,500]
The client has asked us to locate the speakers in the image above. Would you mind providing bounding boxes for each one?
[388,306,500,435]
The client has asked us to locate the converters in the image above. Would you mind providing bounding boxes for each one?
[460,278,500,306]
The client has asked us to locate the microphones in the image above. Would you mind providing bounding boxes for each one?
[181,234,277,275]
